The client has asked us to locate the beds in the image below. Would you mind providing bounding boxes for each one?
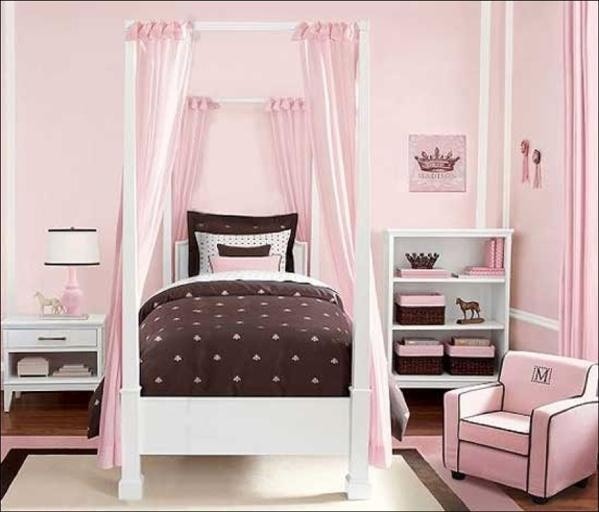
[117,236,374,502]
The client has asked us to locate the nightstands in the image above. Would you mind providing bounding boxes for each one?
[1,314,107,413]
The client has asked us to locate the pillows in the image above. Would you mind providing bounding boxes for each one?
[185,209,299,278]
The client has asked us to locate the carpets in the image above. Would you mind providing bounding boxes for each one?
[0,435,525,512]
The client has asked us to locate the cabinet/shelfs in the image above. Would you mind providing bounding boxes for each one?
[383,227,514,389]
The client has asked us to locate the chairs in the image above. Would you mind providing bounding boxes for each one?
[441,349,598,504]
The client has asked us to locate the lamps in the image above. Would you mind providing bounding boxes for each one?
[43,226,101,317]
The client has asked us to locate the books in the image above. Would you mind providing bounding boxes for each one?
[457,237,505,277]
[52,362,94,376]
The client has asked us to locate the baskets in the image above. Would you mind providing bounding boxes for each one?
[445,341,495,375]
[395,341,445,375]
[395,293,445,324]
